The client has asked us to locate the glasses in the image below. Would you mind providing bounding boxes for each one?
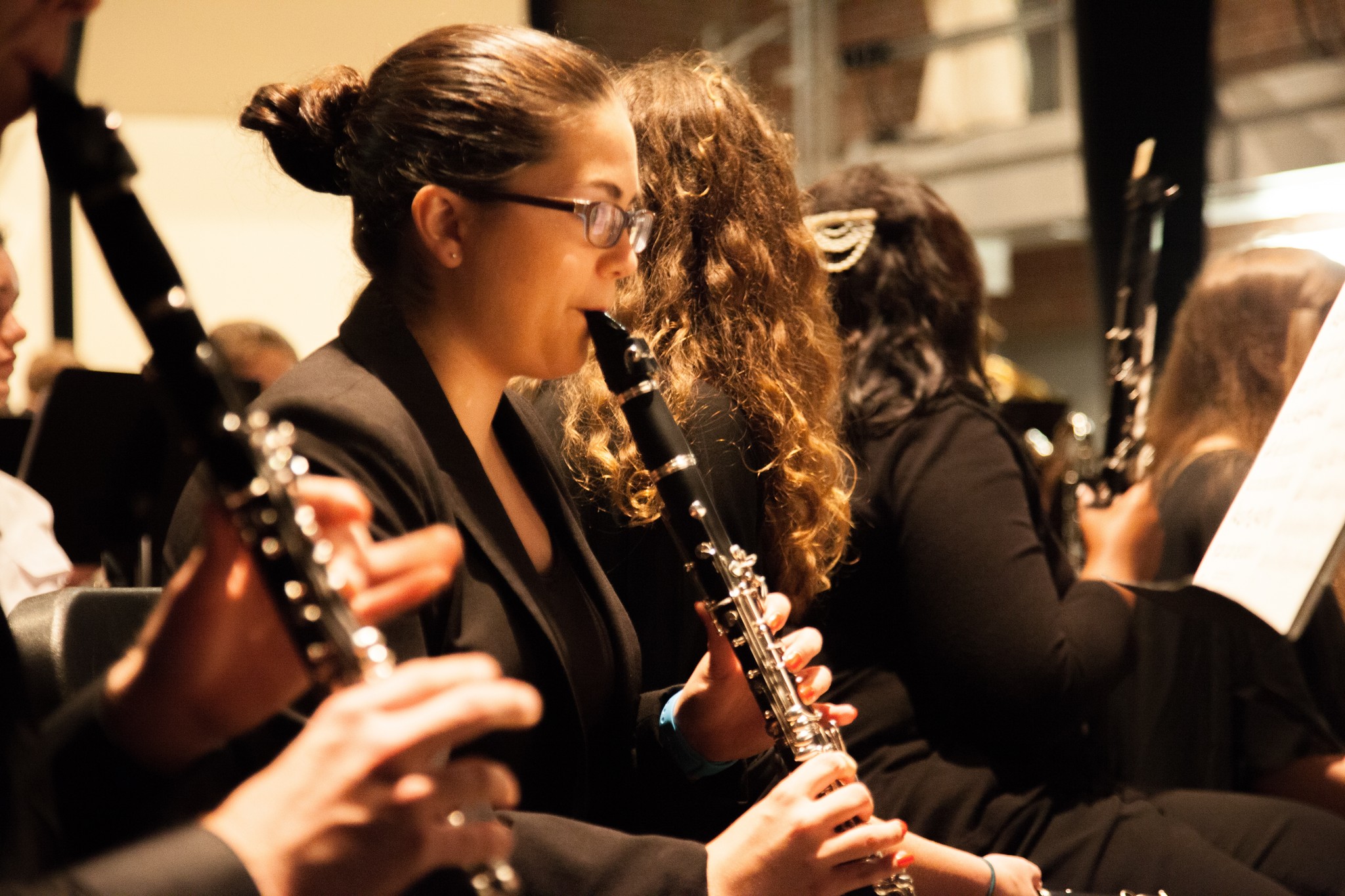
[441,181,655,254]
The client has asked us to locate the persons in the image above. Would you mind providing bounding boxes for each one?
[703,157,1345,896]
[0,333,93,493]
[162,21,920,896]
[509,48,1054,896]
[0,236,154,654]
[0,0,544,896]
[1136,246,1345,799]
[140,314,302,607]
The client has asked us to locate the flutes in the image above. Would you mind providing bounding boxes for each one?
[1065,138,1180,512]
[585,311,921,896]
[27,57,526,896]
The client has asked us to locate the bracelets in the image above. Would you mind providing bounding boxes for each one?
[983,856,996,896]
